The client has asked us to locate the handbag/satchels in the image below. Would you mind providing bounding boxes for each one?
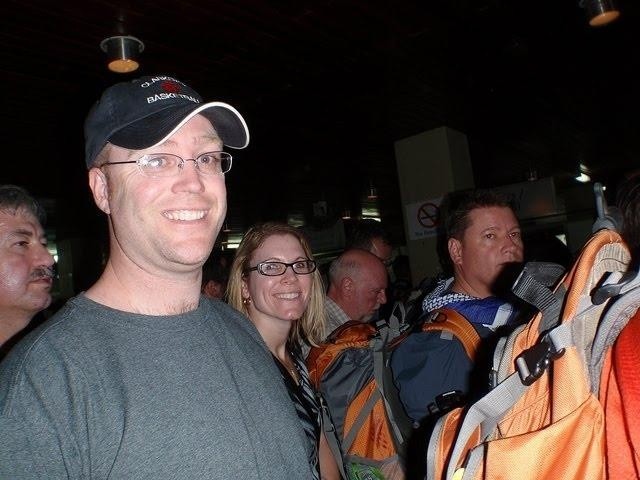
[426,183,640,480]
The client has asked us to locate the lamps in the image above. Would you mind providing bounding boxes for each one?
[578,0,620,27]
[98,12,146,73]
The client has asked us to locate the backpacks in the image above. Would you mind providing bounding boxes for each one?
[306,300,482,480]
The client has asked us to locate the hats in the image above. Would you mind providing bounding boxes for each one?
[85,75,251,169]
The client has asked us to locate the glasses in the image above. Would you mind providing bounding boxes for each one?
[100,151,234,174]
[245,259,317,277]
